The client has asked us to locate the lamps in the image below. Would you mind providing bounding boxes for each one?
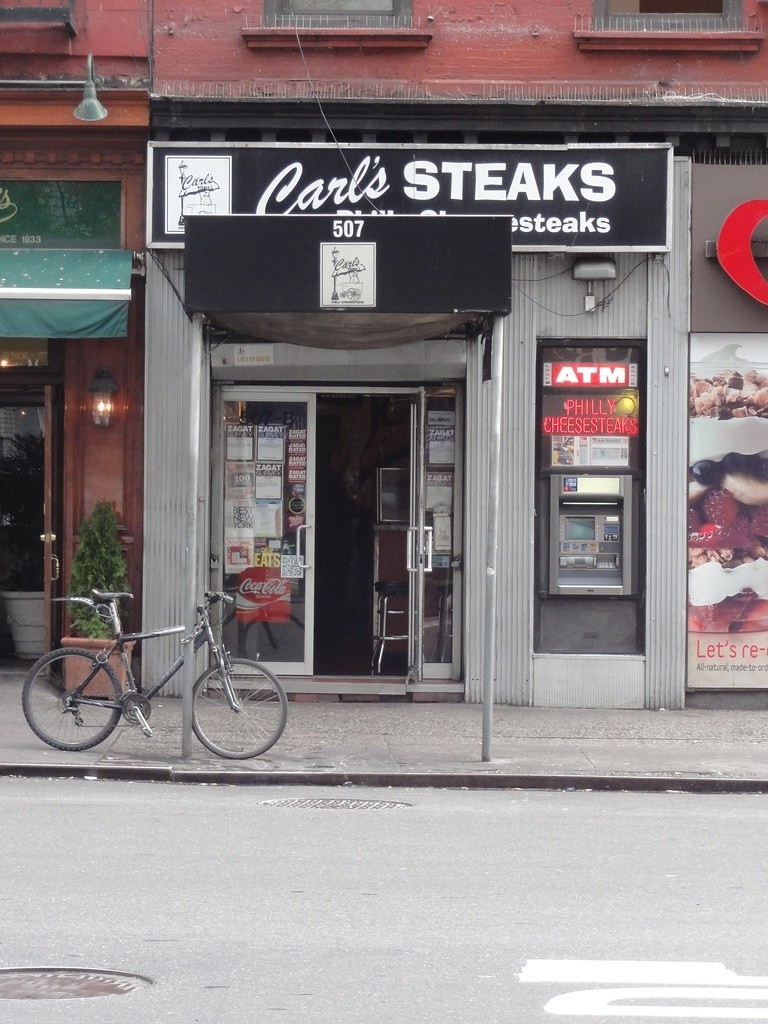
[88,367,119,427]
[72,53,108,121]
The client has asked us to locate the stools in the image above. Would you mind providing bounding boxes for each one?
[372,580,436,676]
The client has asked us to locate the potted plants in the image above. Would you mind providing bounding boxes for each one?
[60,500,137,700]
[0,433,45,659]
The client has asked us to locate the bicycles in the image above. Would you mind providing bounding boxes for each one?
[22,589,290,758]
[224,550,304,664]
